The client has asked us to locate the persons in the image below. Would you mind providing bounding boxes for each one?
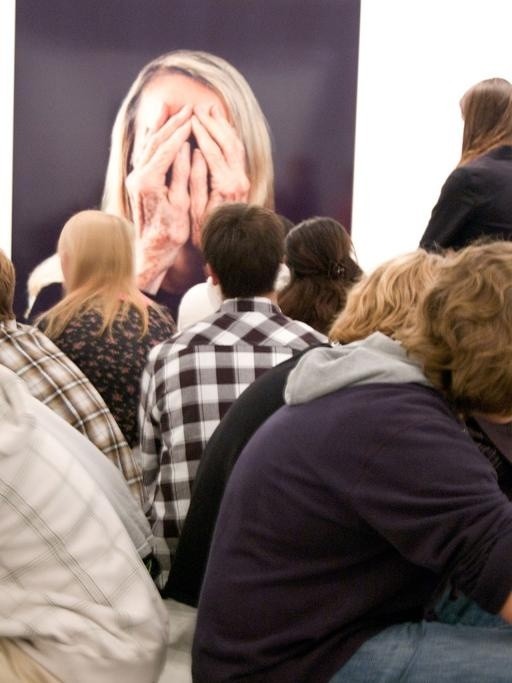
[194,240,512,683]
[415,75,512,250]
[172,251,443,605]
[0,363,171,682]
[22,49,278,322]
[0,210,177,504]
[148,202,365,584]
